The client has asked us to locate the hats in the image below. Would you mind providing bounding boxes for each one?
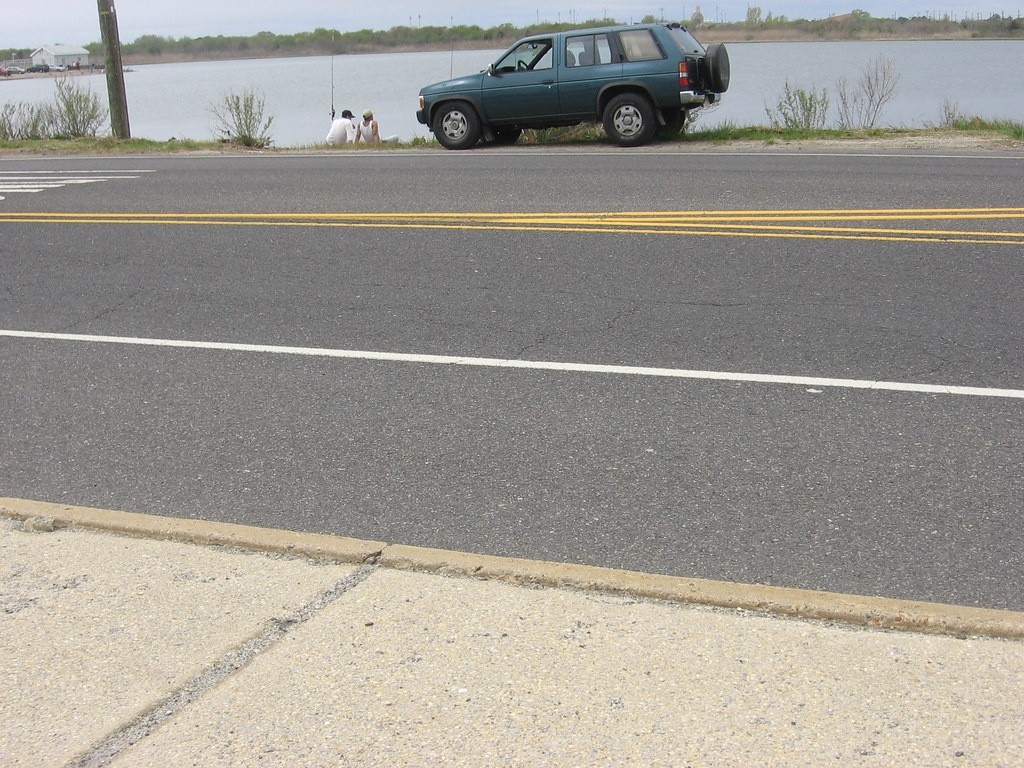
[342,110,356,118]
[362,109,373,118]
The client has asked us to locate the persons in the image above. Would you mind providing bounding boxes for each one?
[354,110,399,145]
[326,110,356,146]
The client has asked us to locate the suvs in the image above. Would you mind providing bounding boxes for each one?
[416,24,731,147]
[6,67,24,74]
[27,64,50,73]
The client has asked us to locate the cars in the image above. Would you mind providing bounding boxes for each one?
[48,64,62,72]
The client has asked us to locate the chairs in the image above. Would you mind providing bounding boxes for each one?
[578,52,586,65]
[567,50,576,66]
